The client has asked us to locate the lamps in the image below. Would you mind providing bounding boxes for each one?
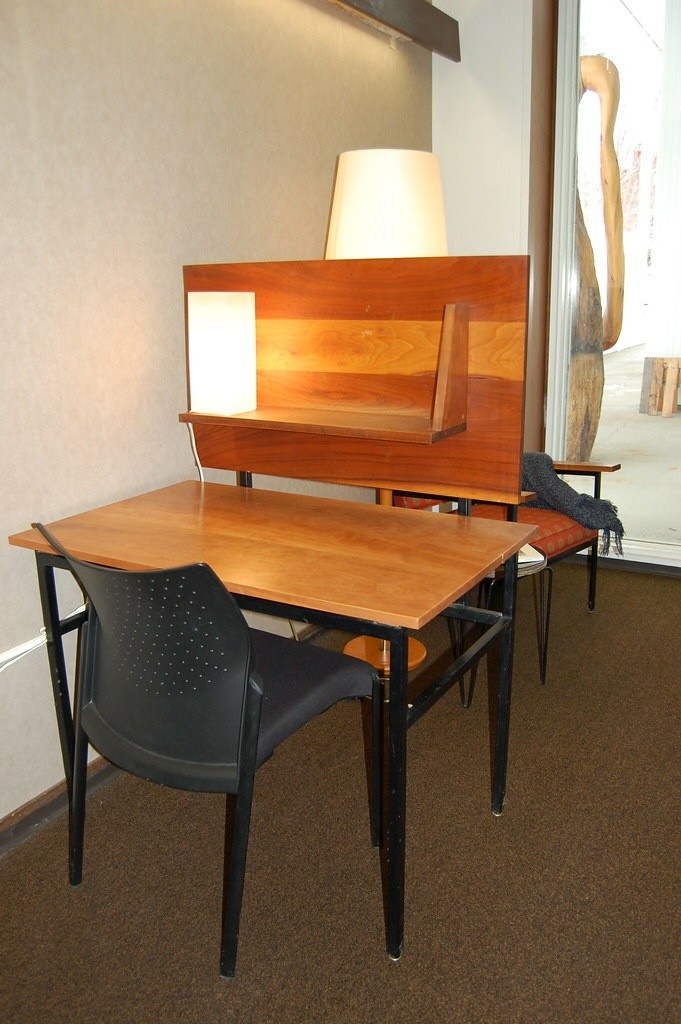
[185,290,258,420]
[323,149,449,677]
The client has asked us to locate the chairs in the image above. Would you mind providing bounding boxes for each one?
[29,520,388,983]
[391,461,622,612]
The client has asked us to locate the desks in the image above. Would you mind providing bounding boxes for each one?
[9,480,539,961]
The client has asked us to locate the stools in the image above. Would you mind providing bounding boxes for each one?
[447,542,554,709]
